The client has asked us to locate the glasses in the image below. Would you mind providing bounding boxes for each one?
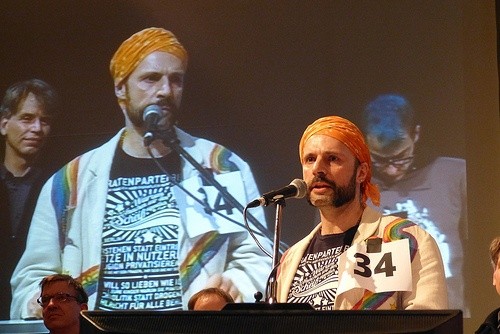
[372,154,415,168]
[37,293,77,305]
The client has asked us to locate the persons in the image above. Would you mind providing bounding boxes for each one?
[188,288,234,311]
[0,75,65,324]
[271,116,448,311]
[37,274,88,334]
[12,26,282,320]
[318,95,470,319]
[475,237,500,334]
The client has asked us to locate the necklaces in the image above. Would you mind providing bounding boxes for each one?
[119,131,127,150]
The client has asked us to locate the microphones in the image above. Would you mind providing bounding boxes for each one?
[143,105,161,146]
[247,179,307,208]
